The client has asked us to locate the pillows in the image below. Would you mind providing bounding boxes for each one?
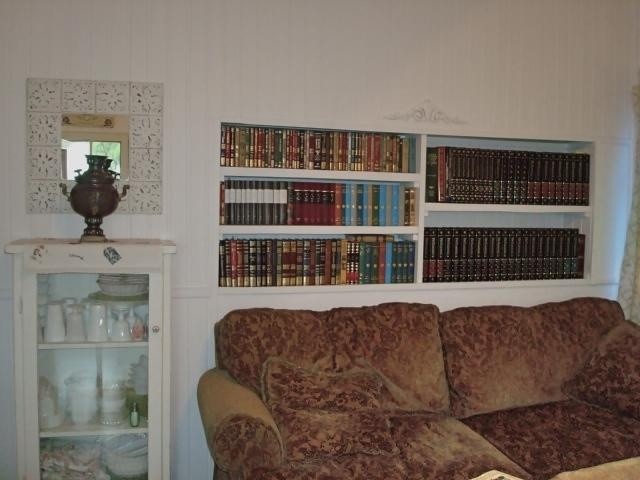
[560,319,639,420]
[261,355,400,466]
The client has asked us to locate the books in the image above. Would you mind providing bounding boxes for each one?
[425,146,590,206]
[220,125,416,174]
[219,176,420,226]
[423,227,586,283]
[219,234,418,288]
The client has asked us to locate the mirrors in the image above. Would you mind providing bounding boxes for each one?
[25,79,164,217]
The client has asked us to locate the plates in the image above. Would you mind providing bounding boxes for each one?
[103,434,147,480]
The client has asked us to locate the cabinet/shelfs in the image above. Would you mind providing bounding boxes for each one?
[211,119,599,296]
[5,238,176,479]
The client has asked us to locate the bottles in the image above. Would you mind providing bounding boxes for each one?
[68,154,120,235]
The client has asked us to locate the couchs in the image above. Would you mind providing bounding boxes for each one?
[199,297,640,479]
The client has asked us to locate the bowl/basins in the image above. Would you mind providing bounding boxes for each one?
[95,274,149,298]
[40,354,148,428]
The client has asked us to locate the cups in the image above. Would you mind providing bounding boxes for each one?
[41,296,148,345]
